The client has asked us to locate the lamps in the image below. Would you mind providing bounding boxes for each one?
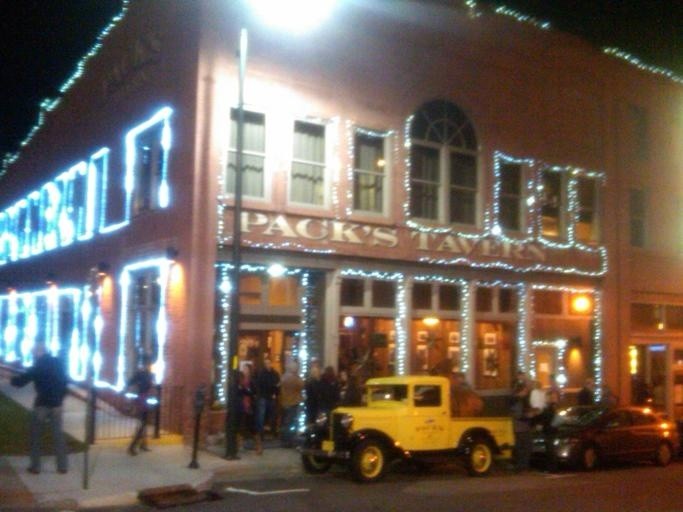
[91,261,107,277]
[6,280,14,295]
[44,272,58,288]
[166,244,179,263]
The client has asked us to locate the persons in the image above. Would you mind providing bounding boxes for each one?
[117,359,158,455]
[225,357,593,456]
[10,345,69,475]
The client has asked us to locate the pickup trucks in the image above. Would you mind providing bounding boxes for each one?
[297,376,517,483]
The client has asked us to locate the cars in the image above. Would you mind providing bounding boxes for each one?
[531,404,681,470]
